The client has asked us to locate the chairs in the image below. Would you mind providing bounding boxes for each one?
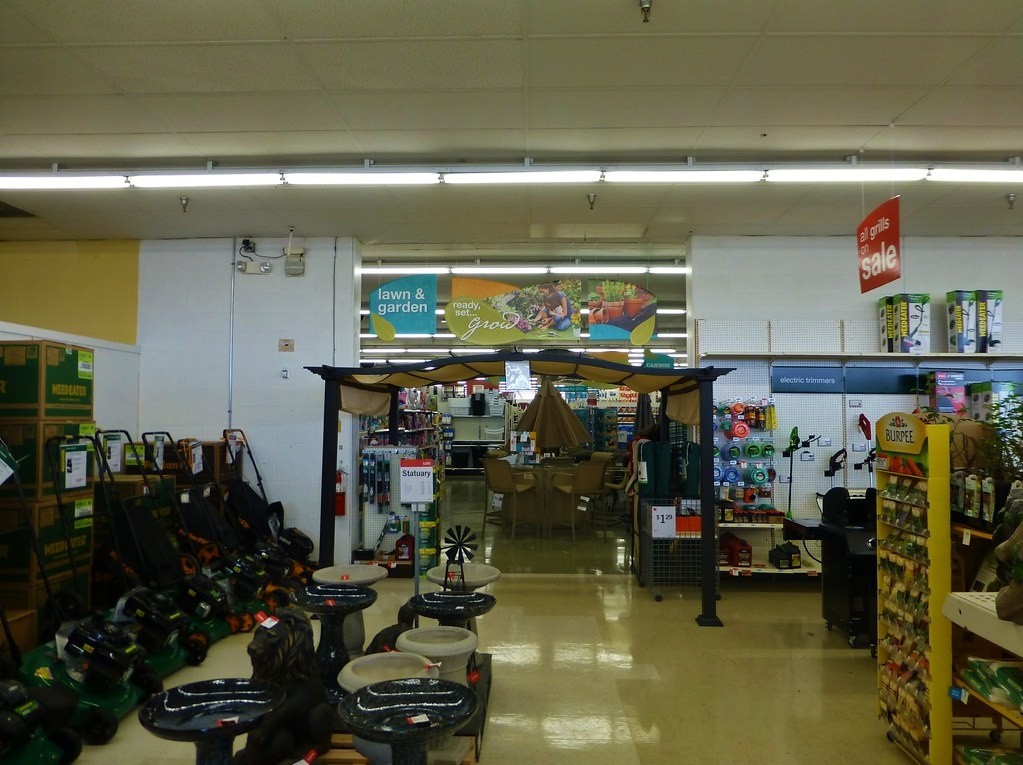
[481,459,543,539]
[590,452,613,462]
[604,461,632,532]
[546,460,608,544]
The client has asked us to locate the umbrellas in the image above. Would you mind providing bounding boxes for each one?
[502,379,592,457]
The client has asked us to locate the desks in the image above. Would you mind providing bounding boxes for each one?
[511,465,577,539]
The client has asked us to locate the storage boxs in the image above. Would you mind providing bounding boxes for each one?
[929,371,965,412]
[417,465,441,576]
[971,382,982,421]
[975,289,1003,354]
[946,289,978,353]
[0,338,245,660]
[448,393,505,416]
[878,295,894,353]
[893,292,931,353]
[982,381,1015,435]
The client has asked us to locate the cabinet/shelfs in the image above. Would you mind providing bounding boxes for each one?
[617,413,658,424]
[941,524,1023,765]
[402,409,439,450]
[716,523,822,574]
[876,468,930,762]
[444,415,505,469]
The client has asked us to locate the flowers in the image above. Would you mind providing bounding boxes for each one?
[482,278,581,338]
[588,279,642,325]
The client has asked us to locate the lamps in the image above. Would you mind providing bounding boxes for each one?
[236,260,270,275]
[359,258,685,275]
[0,156,1023,189]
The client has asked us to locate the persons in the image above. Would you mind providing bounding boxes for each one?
[529,283,574,331]
